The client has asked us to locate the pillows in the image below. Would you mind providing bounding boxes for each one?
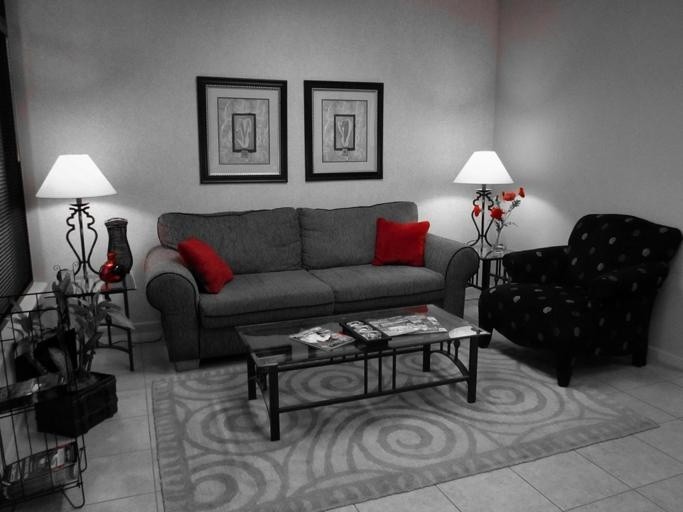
[371,219,429,267]
[178,235,232,293]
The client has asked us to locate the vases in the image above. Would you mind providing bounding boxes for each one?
[493,236,505,255]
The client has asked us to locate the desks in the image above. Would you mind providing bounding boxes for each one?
[55,272,137,372]
[463,244,516,299]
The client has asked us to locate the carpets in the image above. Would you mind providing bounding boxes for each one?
[147,338,661,512]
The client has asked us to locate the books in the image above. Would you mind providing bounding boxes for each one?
[289,326,356,352]
[0,441,79,499]
[402,314,448,334]
[0,371,65,414]
[366,314,421,336]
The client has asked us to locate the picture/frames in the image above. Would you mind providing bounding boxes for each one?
[196,75,288,184]
[303,80,384,183]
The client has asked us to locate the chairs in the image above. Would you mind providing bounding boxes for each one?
[476,214,682,392]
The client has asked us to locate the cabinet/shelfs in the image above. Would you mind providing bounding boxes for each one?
[0,297,96,508]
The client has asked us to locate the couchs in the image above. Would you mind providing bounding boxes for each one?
[144,203,478,370]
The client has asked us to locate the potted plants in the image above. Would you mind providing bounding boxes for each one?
[7,286,137,438]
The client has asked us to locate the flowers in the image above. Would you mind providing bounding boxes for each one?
[472,187,526,232]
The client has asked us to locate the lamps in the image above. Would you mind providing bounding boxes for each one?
[34,153,116,282]
[454,151,514,250]
